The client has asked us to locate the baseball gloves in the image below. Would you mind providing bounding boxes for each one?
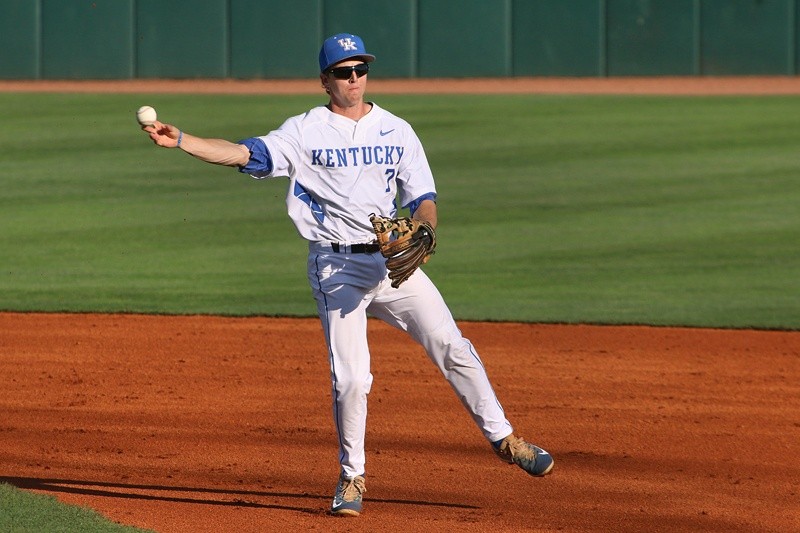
[370,213,437,288]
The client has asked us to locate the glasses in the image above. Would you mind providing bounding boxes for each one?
[324,63,369,79]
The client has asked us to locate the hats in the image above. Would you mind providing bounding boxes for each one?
[319,33,376,74]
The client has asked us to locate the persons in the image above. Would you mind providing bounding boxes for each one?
[142,32,555,516]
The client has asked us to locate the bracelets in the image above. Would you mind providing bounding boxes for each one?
[176,130,183,148]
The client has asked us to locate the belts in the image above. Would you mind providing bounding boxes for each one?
[331,242,381,255]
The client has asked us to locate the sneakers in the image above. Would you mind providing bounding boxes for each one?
[331,472,367,517]
[490,434,554,477]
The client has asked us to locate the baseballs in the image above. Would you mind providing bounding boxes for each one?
[136,106,157,126]
